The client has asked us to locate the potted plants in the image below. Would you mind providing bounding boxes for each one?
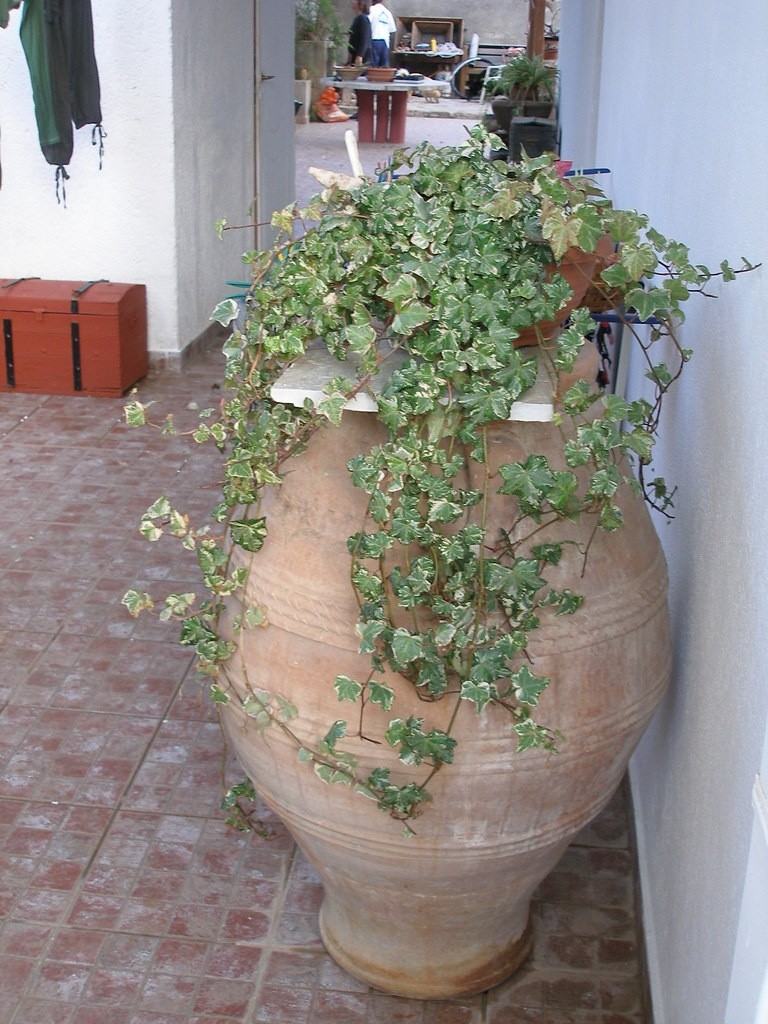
[295,0,358,80]
[486,50,561,129]
[120,120,767,847]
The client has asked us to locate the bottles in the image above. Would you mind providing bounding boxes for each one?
[431,36,437,51]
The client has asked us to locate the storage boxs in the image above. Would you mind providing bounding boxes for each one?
[391,16,466,53]
[0,278,148,398]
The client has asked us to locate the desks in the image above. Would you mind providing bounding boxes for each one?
[318,74,450,144]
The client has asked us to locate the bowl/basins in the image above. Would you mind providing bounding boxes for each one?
[366,66,397,77]
[365,76,396,82]
[332,68,366,80]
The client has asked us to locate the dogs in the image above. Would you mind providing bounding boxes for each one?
[420,89,441,103]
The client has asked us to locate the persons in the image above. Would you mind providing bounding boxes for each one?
[342,0,397,121]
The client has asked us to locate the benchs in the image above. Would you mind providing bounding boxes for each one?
[458,43,529,98]
